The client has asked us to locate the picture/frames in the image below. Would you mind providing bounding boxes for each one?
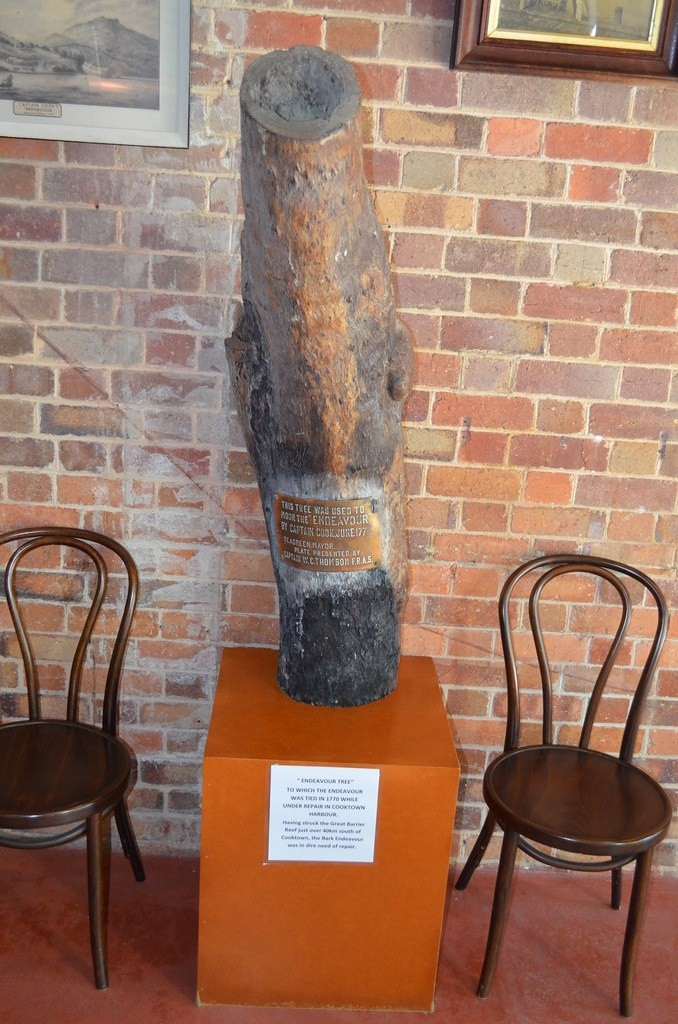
[0,0,191,148]
[449,0,678,90]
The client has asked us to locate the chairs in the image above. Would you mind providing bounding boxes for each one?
[0,525,146,990]
[453,554,673,1017]
